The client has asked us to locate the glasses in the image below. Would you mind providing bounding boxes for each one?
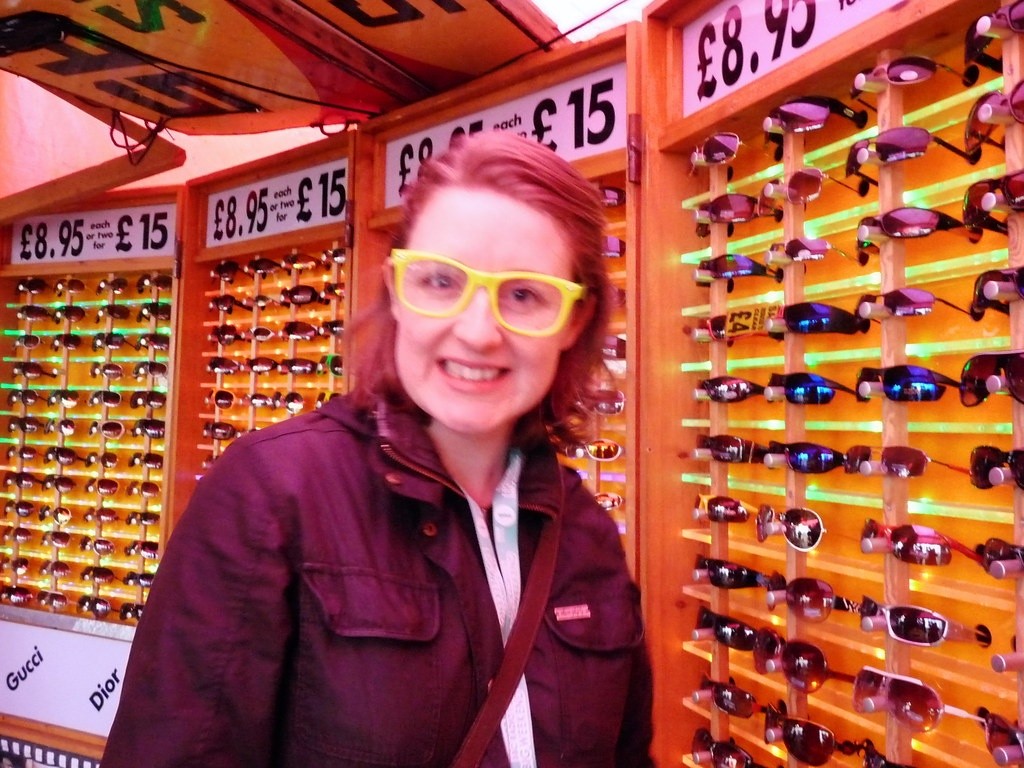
[200,247,346,479]
[684,0,1024,768]
[390,248,591,337]
[0,269,172,625]
[550,185,627,509]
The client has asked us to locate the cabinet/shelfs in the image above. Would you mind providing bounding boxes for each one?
[0,0,1024,768]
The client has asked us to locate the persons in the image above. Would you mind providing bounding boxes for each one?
[99,128,657,767]
[913,387,931,400]
[927,621,940,643]
[798,531,802,539]
[916,547,937,565]
